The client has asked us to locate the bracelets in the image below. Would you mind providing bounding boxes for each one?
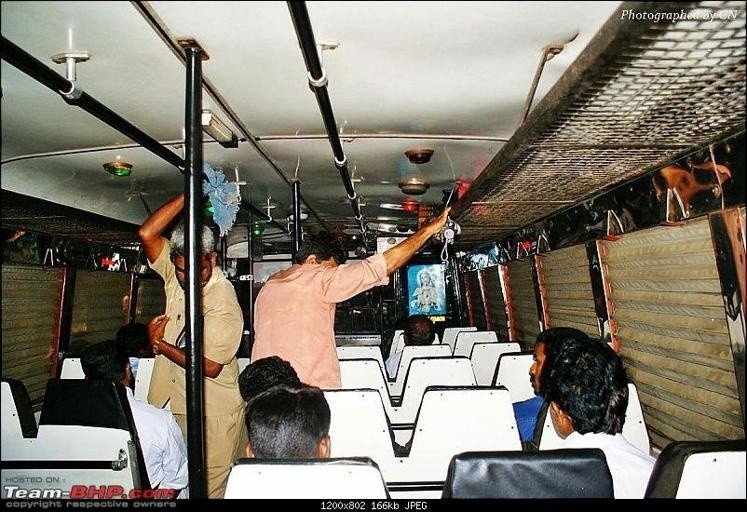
[153,337,161,355]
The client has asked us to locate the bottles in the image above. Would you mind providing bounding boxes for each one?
[251,222,264,261]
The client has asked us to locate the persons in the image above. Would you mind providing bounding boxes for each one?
[114,322,155,358]
[233,353,302,398]
[135,188,251,500]
[238,380,335,459]
[512,325,590,455]
[250,205,453,392]
[76,338,190,499]
[410,269,443,315]
[539,336,661,499]
[385,313,436,378]
